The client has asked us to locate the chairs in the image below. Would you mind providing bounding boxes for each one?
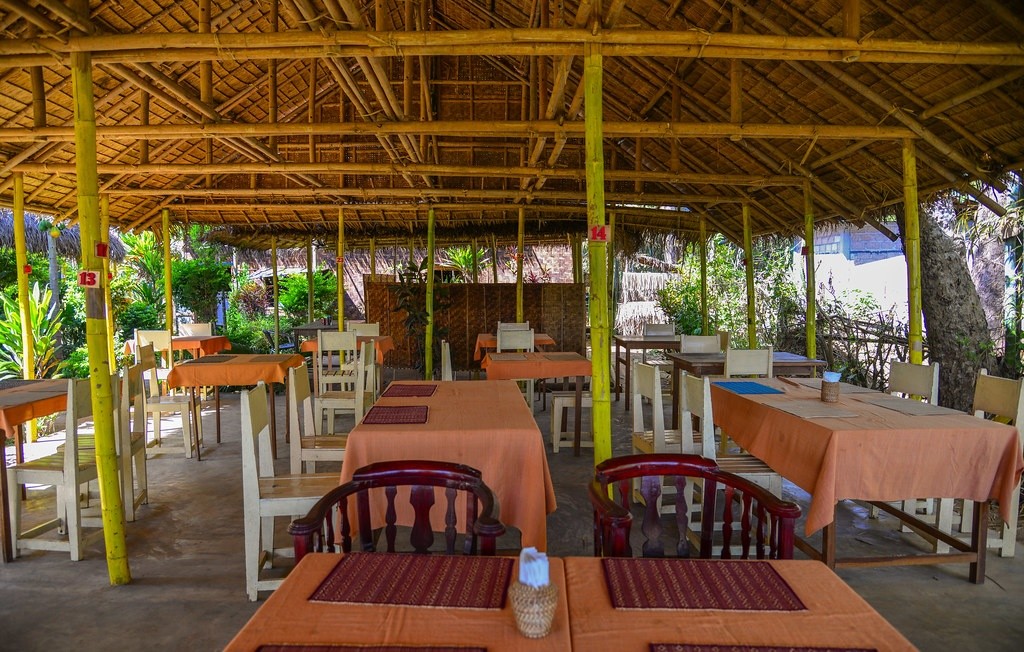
[440,340,454,383]
[586,453,801,559]
[551,384,594,454]
[630,358,738,517]
[841,358,940,518]
[178,322,216,402]
[901,369,1023,558]
[642,322,772,452]
[288,361,351,474]
[289,460,505,563]
[355,340,379,426]
[346,320,382,340]
[316,331,358,438]
[56,329,204,522]
[495,321,535,418]
[240,380,341,600]
[263,330,294,355]
[677,368,782,553]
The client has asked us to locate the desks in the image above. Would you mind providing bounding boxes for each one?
[221,551,572,652]
[0,371,78,564]
[664,349,828,430]
[613,333,682,411]
[168,352,304,459]
[333,380,558,554]
[168,334,232,443]
[289,317,366,355]
[300,335,395,398]
[481,350,593,456]
[473,332,555,411]
[564,555,919,652]
[705,374,1024,585]
